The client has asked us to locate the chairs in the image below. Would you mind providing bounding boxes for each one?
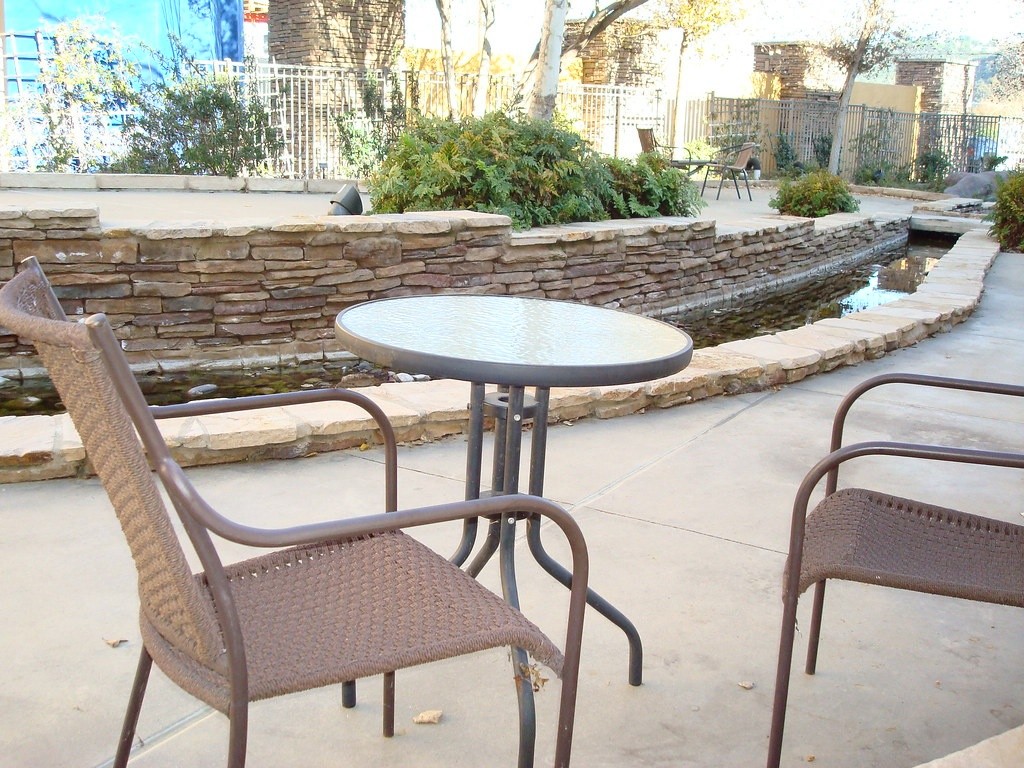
[700,142,755,201]
[767,374,1024,768]
[1,253,589,768]
[636,127,691,177]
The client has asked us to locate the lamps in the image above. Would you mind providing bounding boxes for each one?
[327,184,363,215]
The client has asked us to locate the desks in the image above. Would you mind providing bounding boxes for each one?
[670,159,717,176]
[334,295,693,768]
